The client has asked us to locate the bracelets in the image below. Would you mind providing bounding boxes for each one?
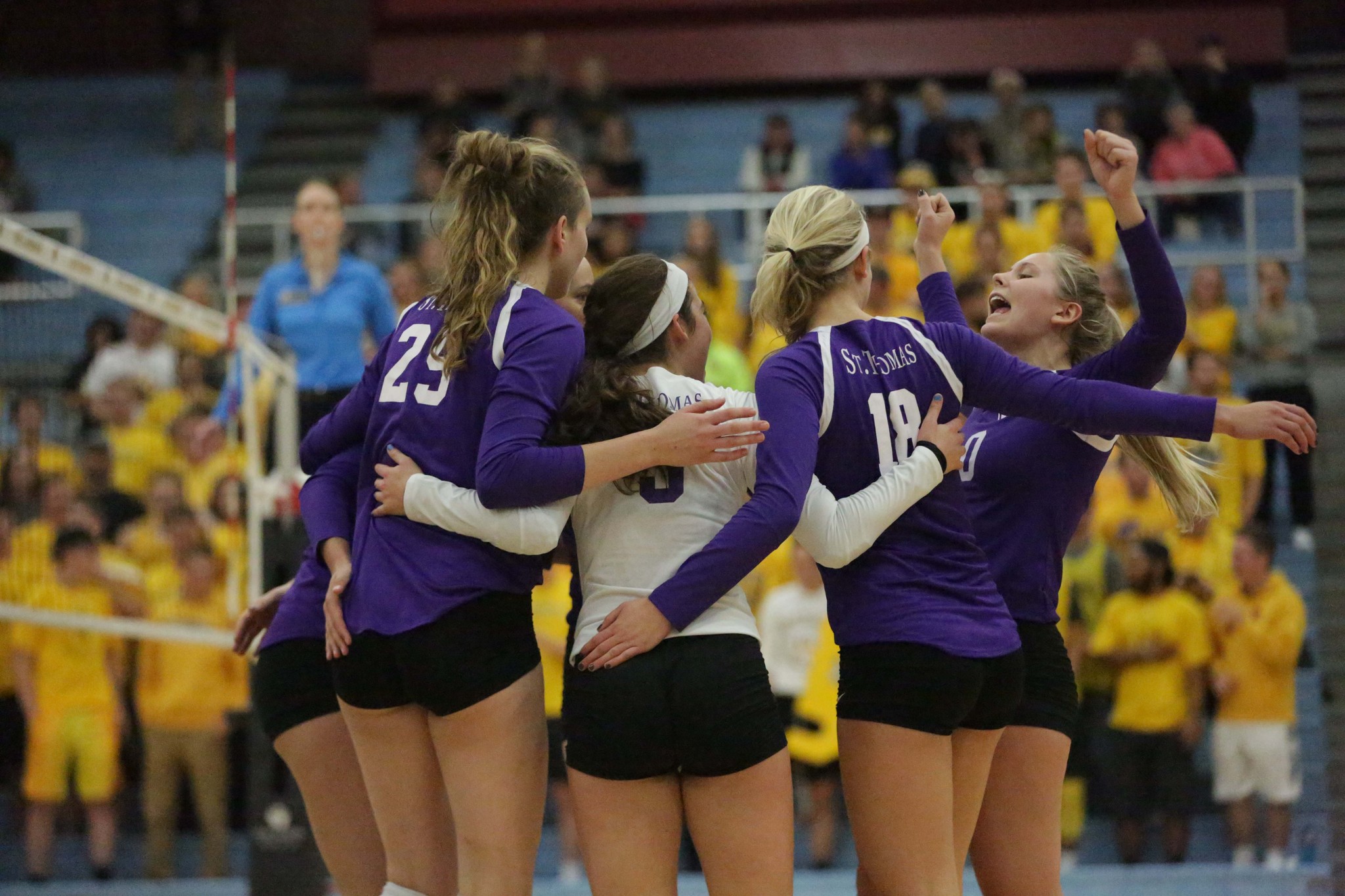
[915,440,948,474]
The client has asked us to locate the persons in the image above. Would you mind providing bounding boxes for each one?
[0,30,1316,896]
[297,125,769,896]
[913,128,1185,896]
[573,183,1324,896]
